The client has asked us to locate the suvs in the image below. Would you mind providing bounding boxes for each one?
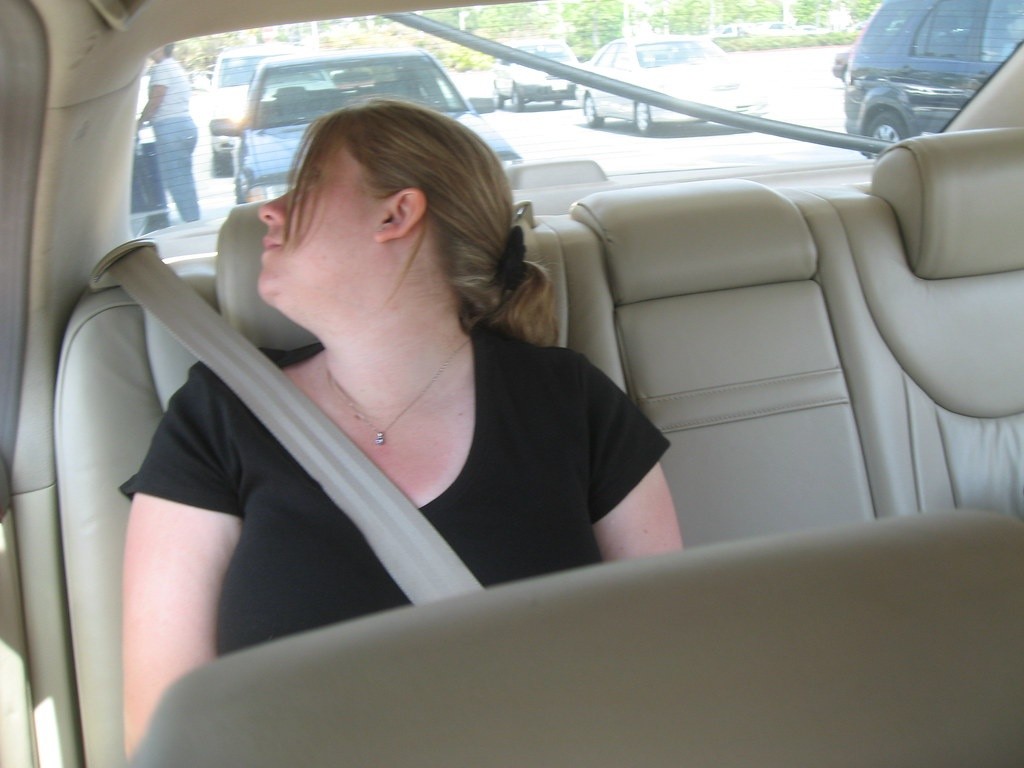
[844,1,1024,161]
[201,39,336,178]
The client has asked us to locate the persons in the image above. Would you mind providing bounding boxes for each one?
[137,44,200,221]
[118,95,686,768]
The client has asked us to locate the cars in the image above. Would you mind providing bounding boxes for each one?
[487,37,584,113]
[208,44,519,206]
[573,34,774,138]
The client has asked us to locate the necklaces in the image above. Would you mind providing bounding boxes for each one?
[324,337,470,448]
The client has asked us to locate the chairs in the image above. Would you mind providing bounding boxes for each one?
[52,139,1022,767]
